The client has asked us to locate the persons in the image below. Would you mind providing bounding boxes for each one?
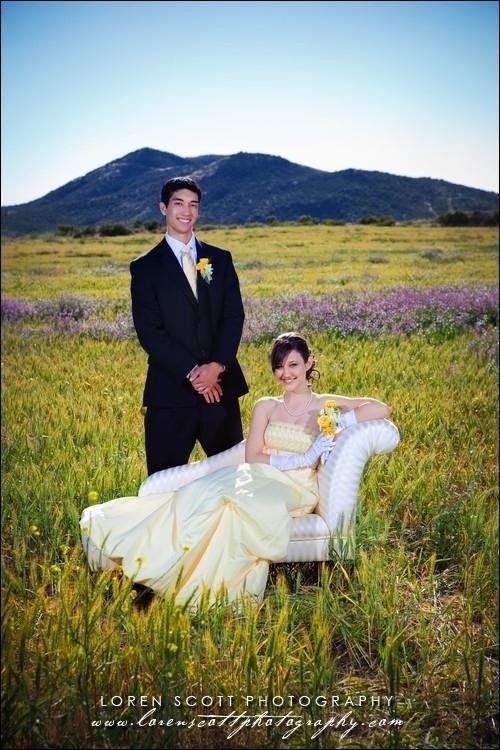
[129,176,251,477]
[79,331,391,617]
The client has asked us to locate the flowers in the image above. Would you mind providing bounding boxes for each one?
[194,257,214,285]
[317,399,340,435]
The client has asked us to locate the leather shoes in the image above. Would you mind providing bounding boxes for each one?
[131,583,154,605]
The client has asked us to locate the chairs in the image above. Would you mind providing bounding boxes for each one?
[137,417,401,563]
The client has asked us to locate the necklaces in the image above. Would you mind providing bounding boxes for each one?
[282,390,312,416]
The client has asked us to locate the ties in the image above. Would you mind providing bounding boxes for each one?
[180,245,198,302]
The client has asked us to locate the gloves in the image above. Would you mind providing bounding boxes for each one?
[320,408,357,465]
[269,432,336,472]
